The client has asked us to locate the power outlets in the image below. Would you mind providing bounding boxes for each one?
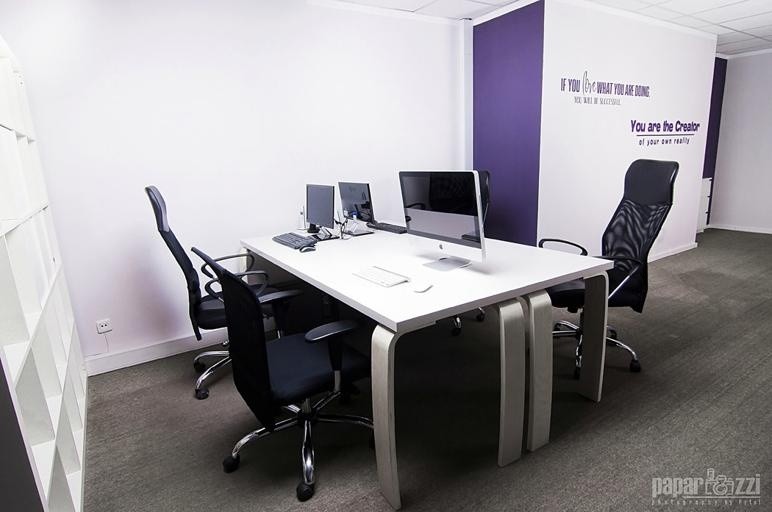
[96,318,113,334]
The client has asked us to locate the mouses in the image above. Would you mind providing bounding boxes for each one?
[300,245,315,252]
[366,220,378,225]
[414,281,433,293]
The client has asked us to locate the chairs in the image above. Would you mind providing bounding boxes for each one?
[146,185,306,400]
[190,245,373,502]
[539,159,679,380]
[429,168,491,336]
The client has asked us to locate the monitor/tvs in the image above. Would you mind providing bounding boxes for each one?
[338,181,375,236]
[305,184,340,242]
[399,170,486,272]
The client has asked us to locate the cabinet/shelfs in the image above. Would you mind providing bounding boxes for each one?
[0,38,88,512]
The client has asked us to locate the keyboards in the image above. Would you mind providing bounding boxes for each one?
[353,264,410,288]
[367,222,407,234]
[273,232,316,249]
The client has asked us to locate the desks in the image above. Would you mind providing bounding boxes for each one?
[240,226,615,511]
